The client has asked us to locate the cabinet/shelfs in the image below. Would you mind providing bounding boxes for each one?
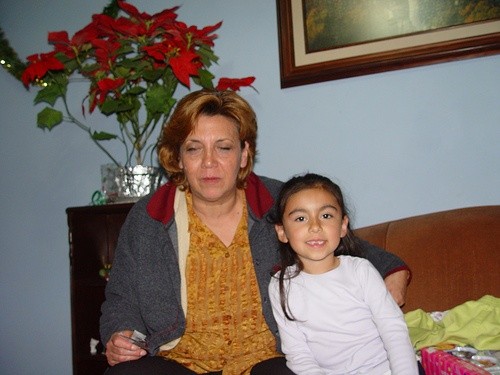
[66,203,133,375]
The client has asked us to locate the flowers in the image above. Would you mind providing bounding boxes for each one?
[0,0,260,167]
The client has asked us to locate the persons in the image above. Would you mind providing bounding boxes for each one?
[100,90,412,375]
[267,171,419,375]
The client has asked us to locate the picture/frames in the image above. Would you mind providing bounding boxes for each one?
[276,0,500,88]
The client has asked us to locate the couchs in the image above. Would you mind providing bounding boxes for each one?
[352,205,500,315]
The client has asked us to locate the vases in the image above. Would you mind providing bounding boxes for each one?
[101,163,162,203]
